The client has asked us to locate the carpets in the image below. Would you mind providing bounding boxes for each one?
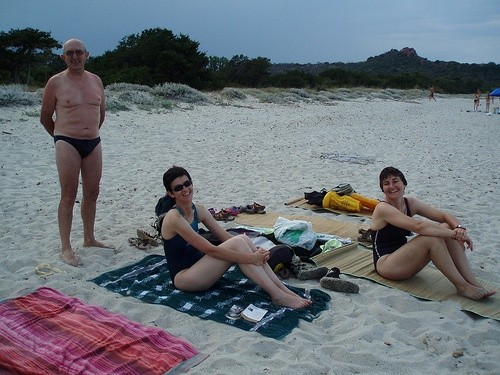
[84,251,335,340]
[0,285,212,375]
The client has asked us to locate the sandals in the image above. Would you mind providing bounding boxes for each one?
[357,228,372,241]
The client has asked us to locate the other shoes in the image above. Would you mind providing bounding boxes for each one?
[207,202,266,221]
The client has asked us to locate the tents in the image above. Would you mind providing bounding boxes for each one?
[489,88,500,96]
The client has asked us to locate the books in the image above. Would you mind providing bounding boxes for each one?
[224,303,268,323]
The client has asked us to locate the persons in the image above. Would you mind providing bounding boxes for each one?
[40,40,117,267]
[473,89,490,112]
[428,85,436,101]
[370,167,496,300]
[160,168,312,310]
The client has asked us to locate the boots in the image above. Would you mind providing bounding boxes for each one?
[320,267,359,293]
[288,260,328,280]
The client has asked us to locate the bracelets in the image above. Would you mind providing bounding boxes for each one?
[453,225,466,230]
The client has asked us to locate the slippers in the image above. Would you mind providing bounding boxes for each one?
[128,229,160,249]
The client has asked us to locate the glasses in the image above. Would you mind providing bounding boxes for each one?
[66,50,86,55]
[172,180,191,191]
